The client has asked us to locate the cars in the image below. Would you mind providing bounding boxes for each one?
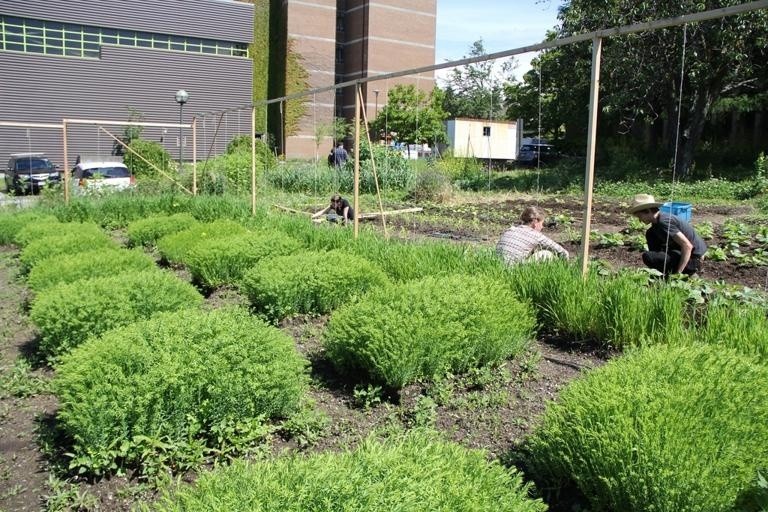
[68,162,138,195]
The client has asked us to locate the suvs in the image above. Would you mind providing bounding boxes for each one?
[520,144,554,162]
[5,154,62,195]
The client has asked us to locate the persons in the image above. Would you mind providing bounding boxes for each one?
[311,194,354,228]
[331,141,347,165]
[328,148,334,166]
[627,192,708,282]
[496,206,570,265]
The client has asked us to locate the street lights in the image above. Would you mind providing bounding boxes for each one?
[175,90,189,166]
[373,89,382,119]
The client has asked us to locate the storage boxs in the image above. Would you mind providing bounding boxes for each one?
[662,201,692,224]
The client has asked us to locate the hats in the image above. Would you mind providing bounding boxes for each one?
[627,194,664,213]
